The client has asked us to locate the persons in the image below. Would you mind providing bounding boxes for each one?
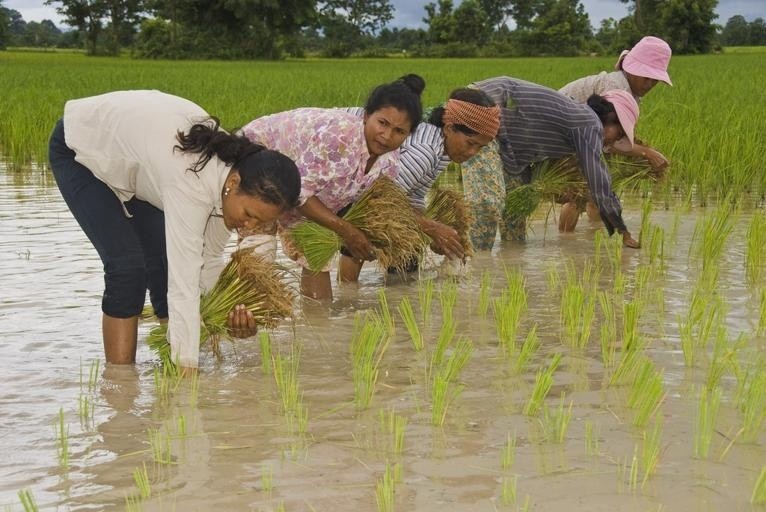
[334,85,501,285]
[221,65,427,308]
[46,86,302,377]
[546,33,675,233]
[452,72,647,260]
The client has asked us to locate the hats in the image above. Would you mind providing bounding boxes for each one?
[622,36,673,88]
[598,89,641,153]
